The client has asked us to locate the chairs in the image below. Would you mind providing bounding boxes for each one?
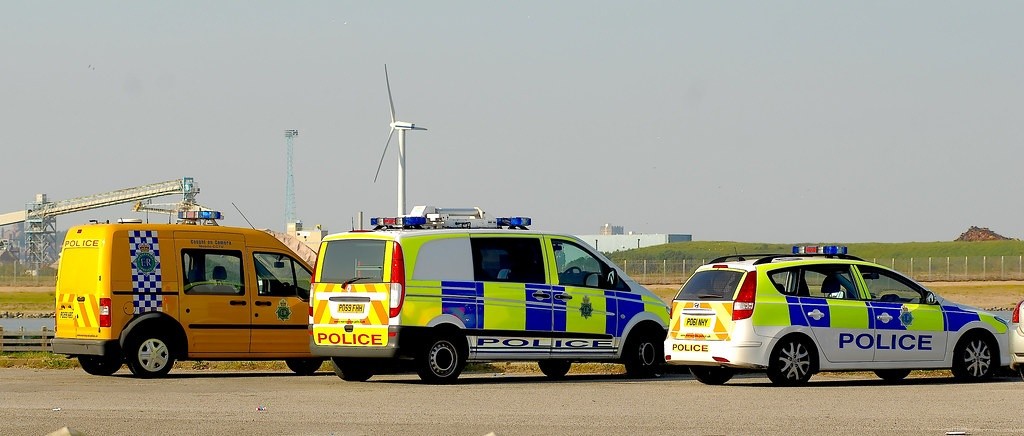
[820,275,841,298]
[188,265,237,293]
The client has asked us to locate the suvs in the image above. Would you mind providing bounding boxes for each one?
[663,245,1011,387]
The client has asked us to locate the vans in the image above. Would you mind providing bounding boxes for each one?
[307,206,669,385]
[52,210,331,379]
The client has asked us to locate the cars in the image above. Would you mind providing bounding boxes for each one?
[1008,300,1024,382]
